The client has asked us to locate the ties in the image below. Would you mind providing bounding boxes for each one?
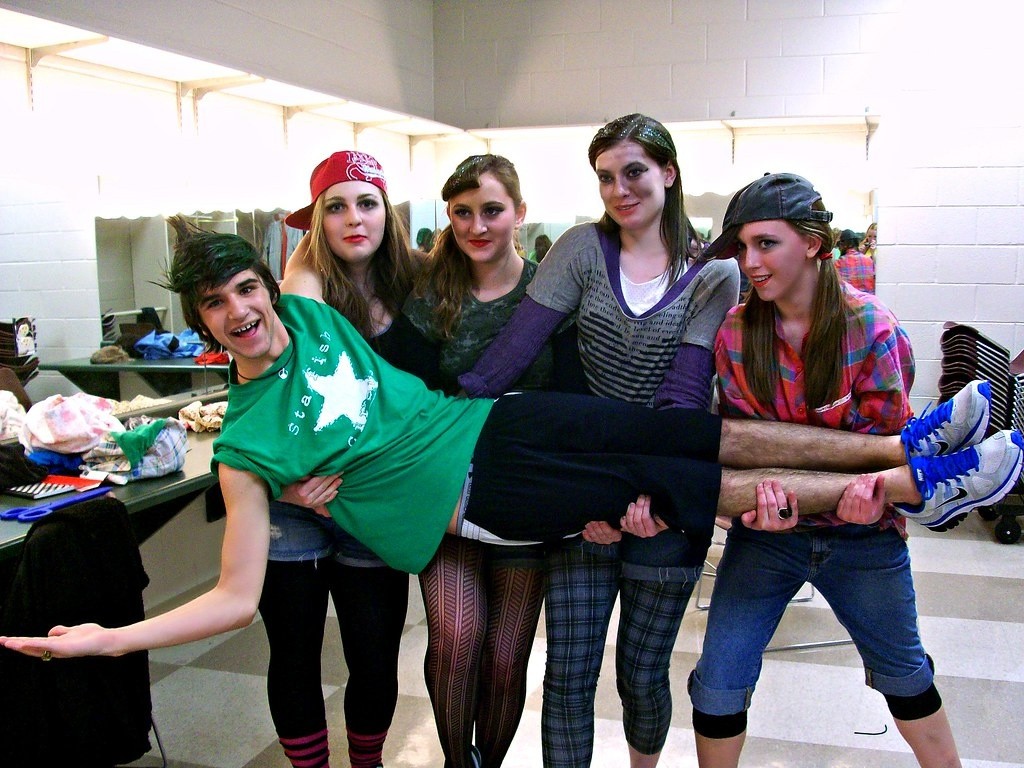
[280,219,288,280]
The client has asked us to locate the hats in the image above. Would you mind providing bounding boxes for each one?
[704,172,833,260]
[285,151,388,230]
[840,229,861,240]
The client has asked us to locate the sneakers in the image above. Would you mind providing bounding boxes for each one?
[892,379,1024,532]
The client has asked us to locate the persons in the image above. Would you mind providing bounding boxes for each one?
[405,155,580,768]
[695,229,711,249]
[830,223,876,297]
[415,229,441,254]
[208,149,433,767]
[686,171,966,768]
[512,230,553,264]
[2,232,1023,660]
[445,113,741,768]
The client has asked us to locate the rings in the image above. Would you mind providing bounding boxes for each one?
[778,507,792,519]
[42,651,52,660]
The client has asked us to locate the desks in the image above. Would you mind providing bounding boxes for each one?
[0,422,221,612]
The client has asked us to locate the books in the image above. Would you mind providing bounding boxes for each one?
[4,469,103,499]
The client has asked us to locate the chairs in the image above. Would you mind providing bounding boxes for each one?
[936,319,1024,484]
[0,495,167,768]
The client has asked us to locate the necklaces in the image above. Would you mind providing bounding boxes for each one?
[237,332,296,380]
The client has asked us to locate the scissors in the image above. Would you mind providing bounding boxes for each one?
[0,485,113,523]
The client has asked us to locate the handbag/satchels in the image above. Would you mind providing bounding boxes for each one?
[0,392,188,486]
